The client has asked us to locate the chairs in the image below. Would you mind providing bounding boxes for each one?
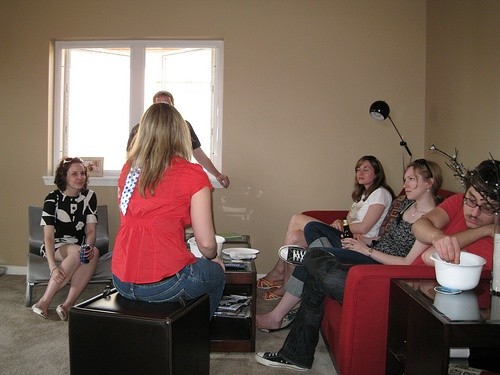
[25,204,113,307]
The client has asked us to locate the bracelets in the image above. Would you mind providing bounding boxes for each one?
[369,247,372,258]
[205,252,218,261]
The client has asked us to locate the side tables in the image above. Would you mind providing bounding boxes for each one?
[385,278,500,375]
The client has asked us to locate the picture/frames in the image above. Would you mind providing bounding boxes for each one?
[80,157,104,177]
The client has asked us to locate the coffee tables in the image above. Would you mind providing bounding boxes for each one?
[208,235,257,353]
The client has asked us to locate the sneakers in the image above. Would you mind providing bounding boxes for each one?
[255,352,309,372]
[278,245,306,266]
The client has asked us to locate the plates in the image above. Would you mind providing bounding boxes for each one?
[221,247,260,255]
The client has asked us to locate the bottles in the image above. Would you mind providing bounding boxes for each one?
[342,216,354,239]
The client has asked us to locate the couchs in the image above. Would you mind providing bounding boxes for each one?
[302,188,492,375]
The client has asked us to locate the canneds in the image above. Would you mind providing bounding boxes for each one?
[80,244,90,264]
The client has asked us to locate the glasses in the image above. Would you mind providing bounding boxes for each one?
[463,189,500,216]
[63,157,84,167]
[414,158,433,178]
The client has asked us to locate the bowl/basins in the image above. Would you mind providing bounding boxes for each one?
[187,234,226,258]
[430,249,487,291]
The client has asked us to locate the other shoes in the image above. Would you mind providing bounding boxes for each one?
[266,307,300,332]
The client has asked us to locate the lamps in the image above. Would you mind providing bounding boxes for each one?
[370,100,412,166]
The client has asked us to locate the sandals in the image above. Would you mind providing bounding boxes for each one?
[55,305,67,321]
[31,304,48,319]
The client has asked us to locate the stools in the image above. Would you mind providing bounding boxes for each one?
[69,288,210,375]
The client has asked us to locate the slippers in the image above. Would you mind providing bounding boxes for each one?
[262,290,284,300]
[256,277,283,289]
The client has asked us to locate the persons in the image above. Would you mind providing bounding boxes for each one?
[50,265,60,276]
[256,155,396,302]
[29,158,101,322]
[255,160,500,372]
[255,159,443,331]
[111,102,226,319]
[127,90,230,190]
